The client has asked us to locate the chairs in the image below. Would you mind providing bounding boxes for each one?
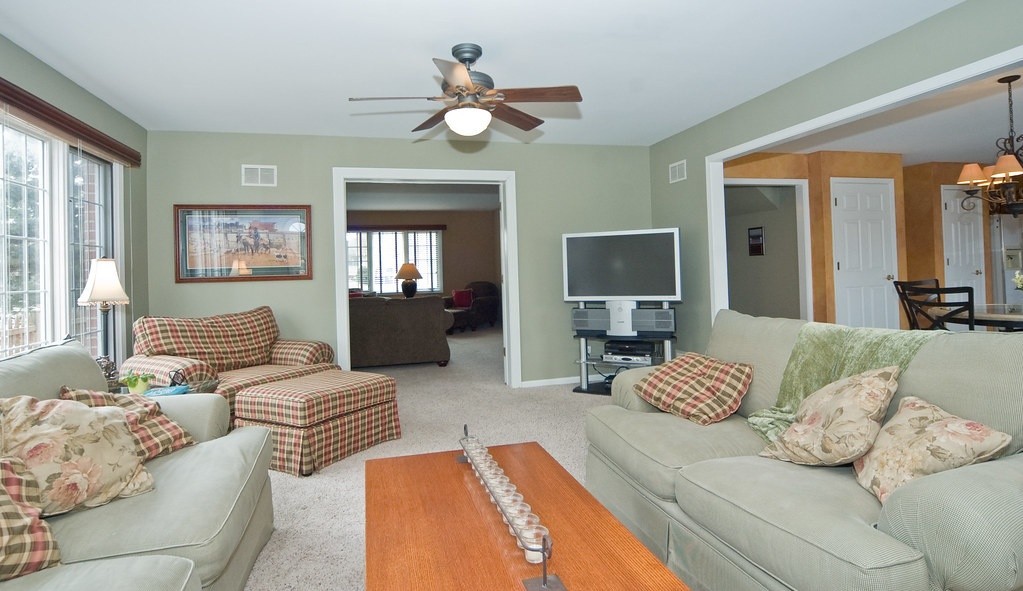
[901,285,975,331]
[893,279,944,330]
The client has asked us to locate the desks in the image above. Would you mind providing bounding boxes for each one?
[927,304,1023,332]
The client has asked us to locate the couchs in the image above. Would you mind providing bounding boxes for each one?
[568,260,1023,591]
[119,304,341,435]
[0,334,275,591]
[441,281,500,332]
[349,295,450,369]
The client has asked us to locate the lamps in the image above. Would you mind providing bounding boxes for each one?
[395,264,423,298]
[76,255,129,394]
[443,103,492,137]
[958,75,1023,218]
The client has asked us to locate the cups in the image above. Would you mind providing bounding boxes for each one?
[520,524,550,564]
[460,436,523,524]
[505,501,531,536]
[513,513,540,548]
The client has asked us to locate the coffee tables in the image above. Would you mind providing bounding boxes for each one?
[366,441,692,591]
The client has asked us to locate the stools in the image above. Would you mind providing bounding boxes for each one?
[444,308,468,335]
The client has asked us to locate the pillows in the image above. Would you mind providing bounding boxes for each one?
[632,351,753,425]
[0,398,155,518]
[850,396,1013,505]
[0,457,62,582]
[452,289,473,308]
[59,384,199,462]
[757,366,903,466]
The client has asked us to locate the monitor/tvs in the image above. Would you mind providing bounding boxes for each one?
[562,228,681,301]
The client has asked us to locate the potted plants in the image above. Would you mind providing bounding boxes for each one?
[118,369,155,394]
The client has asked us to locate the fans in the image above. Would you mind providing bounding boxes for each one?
[347,43,582,133]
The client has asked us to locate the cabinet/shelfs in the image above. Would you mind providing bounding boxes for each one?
[573,335,677,397]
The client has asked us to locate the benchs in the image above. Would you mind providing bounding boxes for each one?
[234,368,402,477]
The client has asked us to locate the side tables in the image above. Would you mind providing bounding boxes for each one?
[119,378,220,394]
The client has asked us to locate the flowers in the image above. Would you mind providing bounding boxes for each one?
[1011,271,1023,291]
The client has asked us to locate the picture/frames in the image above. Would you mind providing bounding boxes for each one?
[173,204,313,283]
